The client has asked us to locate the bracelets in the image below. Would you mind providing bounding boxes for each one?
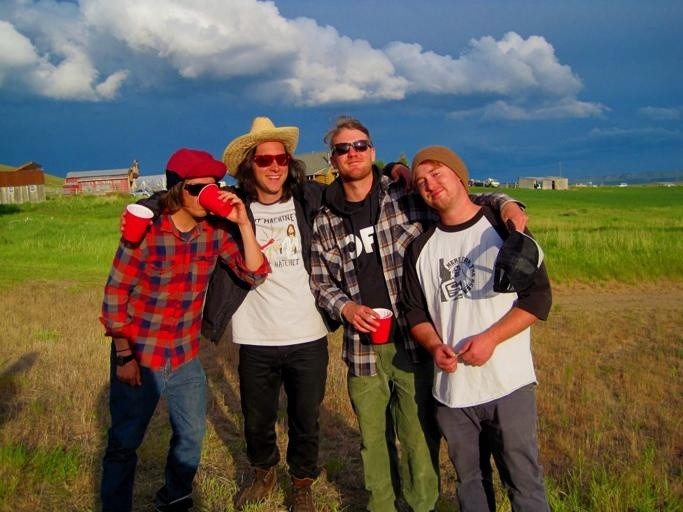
[116,348,130,352]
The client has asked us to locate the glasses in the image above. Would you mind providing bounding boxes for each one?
[332,140,371,154]
[253,151,290,167]
[184,183,221,196]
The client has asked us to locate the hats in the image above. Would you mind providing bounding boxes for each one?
[222,117,299,178]
[166,149,225,189]
[494,220,544,292]
[412,146,469,191]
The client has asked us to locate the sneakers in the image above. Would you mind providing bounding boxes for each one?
[290,475,315,512]
[236,467,278,511]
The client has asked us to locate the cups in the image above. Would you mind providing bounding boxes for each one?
[368,307,393,346]
[197,183,234,219]
[121,204,155,245]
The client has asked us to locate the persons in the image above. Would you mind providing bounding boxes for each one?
[401,146,553,511]
[120,116,412,511]
[309,117,529,511]
[101,149,270,511]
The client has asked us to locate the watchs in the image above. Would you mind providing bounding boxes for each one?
[116,354,135,366]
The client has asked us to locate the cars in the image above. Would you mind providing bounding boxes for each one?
[128,185,153,198]
[616,182,628,188]
[466,178,500,190]
